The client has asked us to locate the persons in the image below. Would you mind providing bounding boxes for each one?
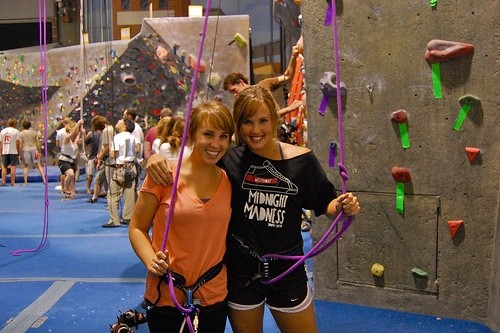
[56,108,195,228]
[19,121,46,184]
[223,45,311,232]
[146,86,360,333]
[129,100,232,333]
[0,119,20,186]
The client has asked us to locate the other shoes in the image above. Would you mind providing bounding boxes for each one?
[120,219,131,225]
[102,222,121,227]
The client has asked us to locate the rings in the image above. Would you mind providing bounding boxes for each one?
[349,208,351,213]
